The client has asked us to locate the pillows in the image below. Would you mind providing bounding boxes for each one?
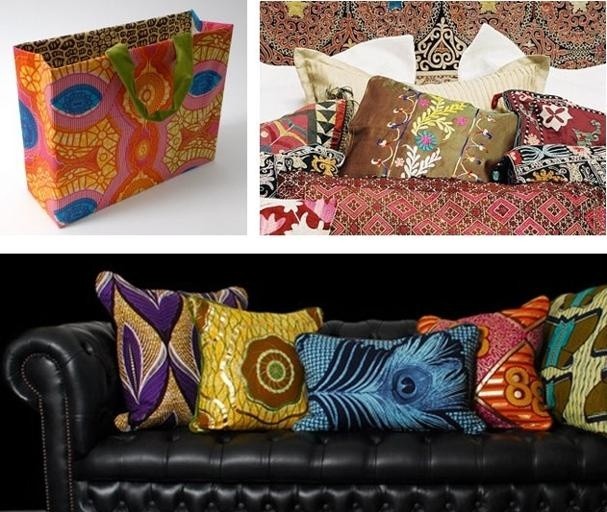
[94,271,607,443]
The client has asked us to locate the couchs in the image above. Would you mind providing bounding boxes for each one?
[4,322,607,512]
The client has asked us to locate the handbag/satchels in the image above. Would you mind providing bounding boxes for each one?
[13,10,234,229]
